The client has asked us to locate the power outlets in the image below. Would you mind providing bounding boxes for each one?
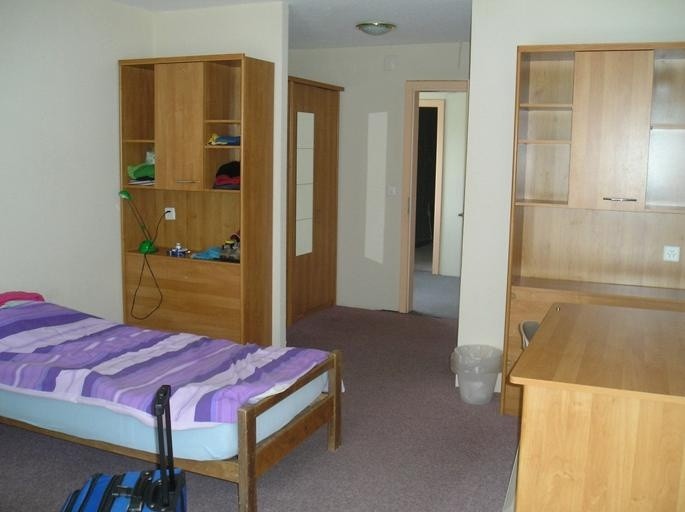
[663,246,681,263]
[163,207,177,221]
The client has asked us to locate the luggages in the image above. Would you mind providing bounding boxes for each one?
[58,384,188,512]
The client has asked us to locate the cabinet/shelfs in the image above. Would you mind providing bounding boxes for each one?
[117,53,275,346]
[500,42,684,416]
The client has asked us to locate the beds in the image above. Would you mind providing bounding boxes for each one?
[0,292,343,512]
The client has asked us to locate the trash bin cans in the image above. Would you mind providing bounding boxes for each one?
[454,344,502,405]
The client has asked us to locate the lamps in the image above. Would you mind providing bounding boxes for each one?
[116,191,163,254]
[355,22,397,36]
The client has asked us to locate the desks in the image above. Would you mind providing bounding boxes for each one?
[507,302,684,512]
